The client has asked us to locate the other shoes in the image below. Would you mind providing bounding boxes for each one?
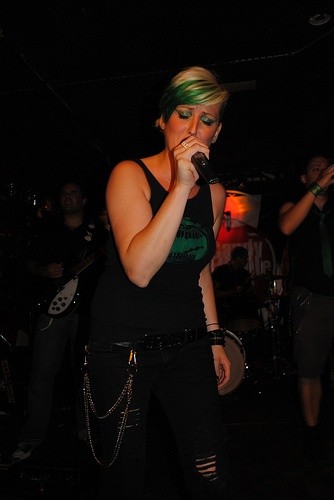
[2,446,33,470]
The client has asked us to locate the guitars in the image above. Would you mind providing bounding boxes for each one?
[30,240,111,320]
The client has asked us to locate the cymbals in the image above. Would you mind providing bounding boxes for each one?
[247,274,288,281]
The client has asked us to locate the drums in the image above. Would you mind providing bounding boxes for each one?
[211,330,247,394]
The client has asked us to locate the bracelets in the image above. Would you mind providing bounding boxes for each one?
[205,323,226,346]
[309,181,323,197]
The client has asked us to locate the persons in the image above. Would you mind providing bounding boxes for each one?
[81,65,236,500]
[210,245,255,331]
[278,156,334,461]
[1,180,110,469]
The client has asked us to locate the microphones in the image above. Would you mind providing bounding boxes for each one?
[191,151,220,185]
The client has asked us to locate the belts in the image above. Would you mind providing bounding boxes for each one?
[107,326,202,355]
